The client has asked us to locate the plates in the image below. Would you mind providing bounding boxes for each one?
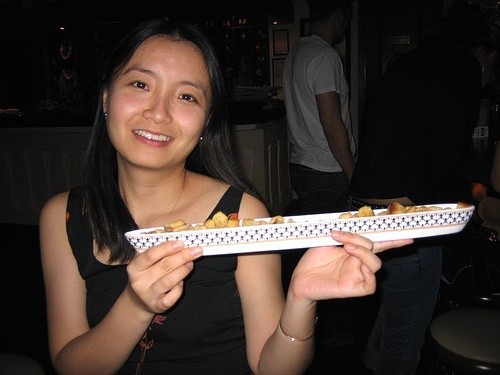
[125,204,476,258]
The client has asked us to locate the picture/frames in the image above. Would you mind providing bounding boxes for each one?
[273,29,290,55]
[272,57,286,89]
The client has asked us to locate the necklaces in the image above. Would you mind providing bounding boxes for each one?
[136,166,186,229]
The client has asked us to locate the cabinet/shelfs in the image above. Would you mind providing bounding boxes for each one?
[263,119,291,215]
[222,125,264,216]
[201,15,265,51]
[0,133,97,226]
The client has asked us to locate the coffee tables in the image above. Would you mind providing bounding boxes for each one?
[425,308,500,375]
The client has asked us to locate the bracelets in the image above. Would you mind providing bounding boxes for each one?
[279,312,317,342]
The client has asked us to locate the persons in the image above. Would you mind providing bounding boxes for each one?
[345,15,500,375]
[282,0,356,346]
[39,13,415,375]
